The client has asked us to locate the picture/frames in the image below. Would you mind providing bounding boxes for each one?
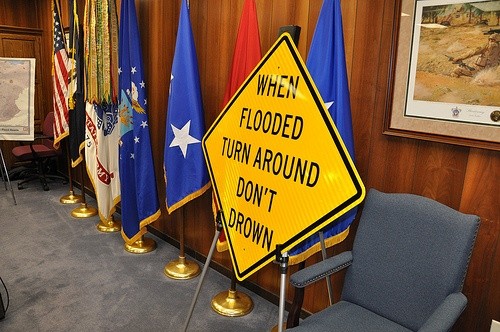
[381,0,500,152]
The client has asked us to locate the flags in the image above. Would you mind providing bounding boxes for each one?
[210,0,262,318]
[52,0,81,205]
[83,0,121,233]
[119,0,161,253]
[163,0,210,280]
[271,0,355,332]
[69,0,96,218]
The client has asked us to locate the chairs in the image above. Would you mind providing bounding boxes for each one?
[277,188,480,332]
[12,113,67,191]
